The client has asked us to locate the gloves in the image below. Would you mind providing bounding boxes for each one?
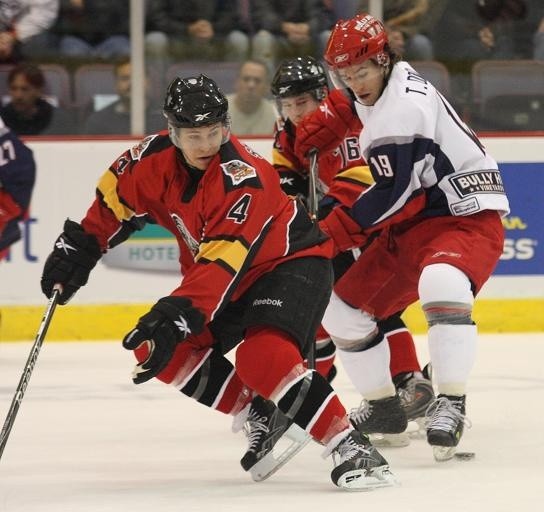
[319,204,367,254]
[123,296,205,384]
[295,89,357,166]
[41,217,102,304]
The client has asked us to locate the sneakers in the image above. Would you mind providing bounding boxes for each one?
[233,364,465,486]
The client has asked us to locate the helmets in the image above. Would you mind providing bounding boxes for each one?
[270,14,391,101]
[163,73,230,149]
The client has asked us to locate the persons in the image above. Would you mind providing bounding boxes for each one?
[1,0,544,263]
[39,72,390,488]
[271,53,438,435]
[316,11,512,447]
